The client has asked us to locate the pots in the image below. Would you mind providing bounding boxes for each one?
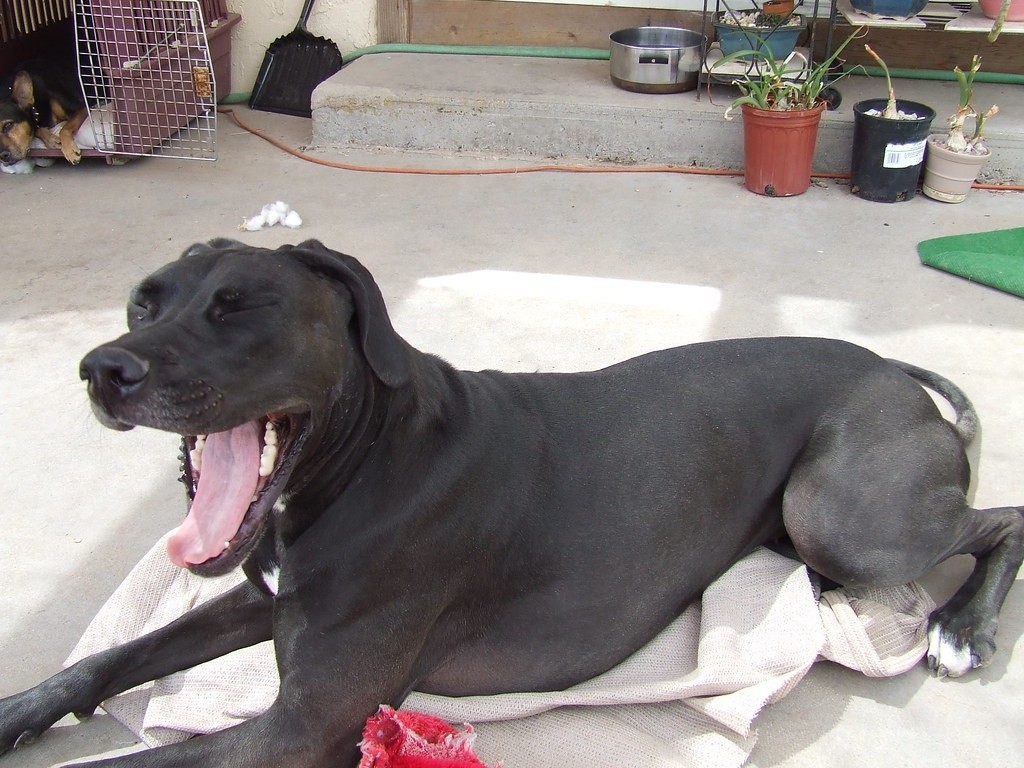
[608,26,708,95]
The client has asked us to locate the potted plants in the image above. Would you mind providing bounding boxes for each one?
[711,13,807,61]
[923,56,1002,204]
[763,0,795,17]
[708,21,869,197]
[853,44,936,202]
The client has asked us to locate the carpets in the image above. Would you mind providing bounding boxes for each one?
[917,228,1024,295]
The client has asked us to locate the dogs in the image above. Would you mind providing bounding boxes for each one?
[0,236,1024,768]
[0,12,114,167]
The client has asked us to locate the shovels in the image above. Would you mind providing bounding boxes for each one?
[248,0,343,118]
